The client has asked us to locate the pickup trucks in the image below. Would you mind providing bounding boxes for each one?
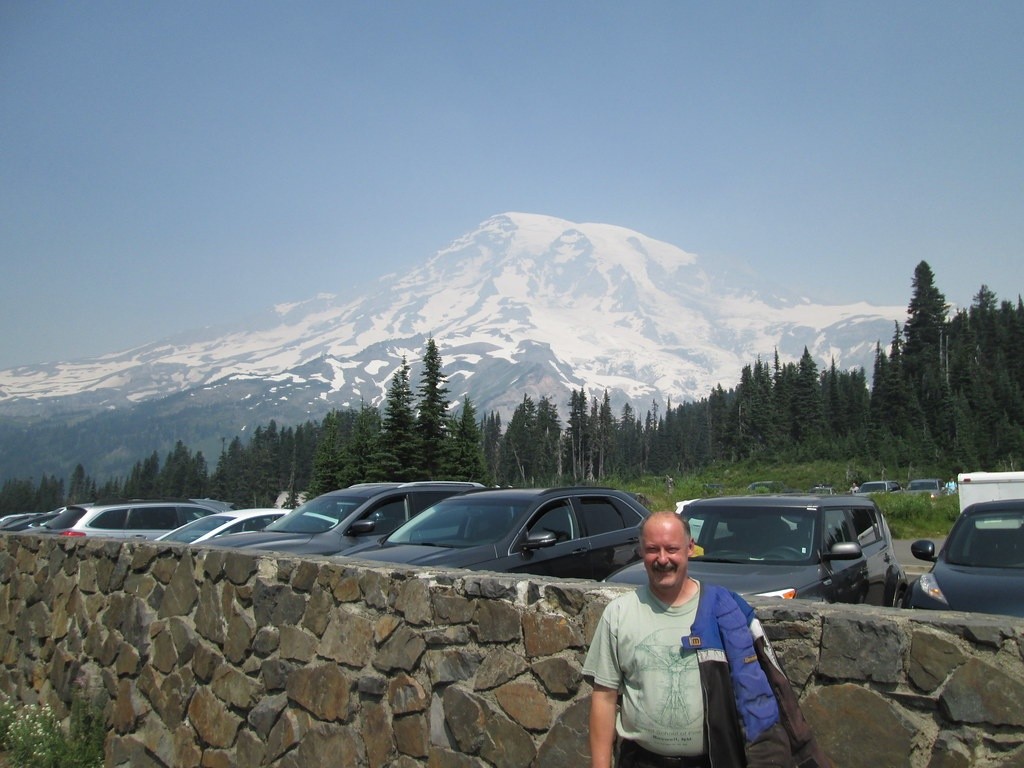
[746,480,805,495]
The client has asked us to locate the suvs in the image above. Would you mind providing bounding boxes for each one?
[331,484,650,583]
[188,478,488,556]
[0,496,240,542]
[597,492,913,612]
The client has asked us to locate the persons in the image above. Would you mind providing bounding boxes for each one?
[581,511,833,768]
[850,482,859,495]
[946,477,957,495]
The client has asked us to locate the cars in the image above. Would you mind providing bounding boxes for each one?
[152,505,343,544]
[903,478,947,500]
[901,497,1024,618]
[850,479,903,497]
[809,486,838,494]
